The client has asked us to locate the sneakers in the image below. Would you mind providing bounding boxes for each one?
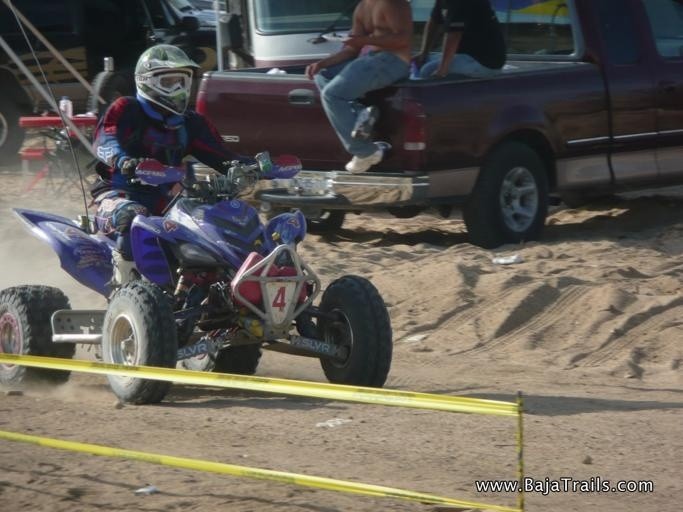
[345,147,382,174]
[351,106,380,142]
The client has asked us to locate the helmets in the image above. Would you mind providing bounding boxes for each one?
[134,44,203,116]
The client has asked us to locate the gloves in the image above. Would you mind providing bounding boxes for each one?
[121,157,153,177]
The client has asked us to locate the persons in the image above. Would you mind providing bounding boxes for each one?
[305,0,413,174]
[411,0,505,81]
[84,43,275,284]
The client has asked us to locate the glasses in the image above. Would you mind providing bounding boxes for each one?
[152,72,194,98]
